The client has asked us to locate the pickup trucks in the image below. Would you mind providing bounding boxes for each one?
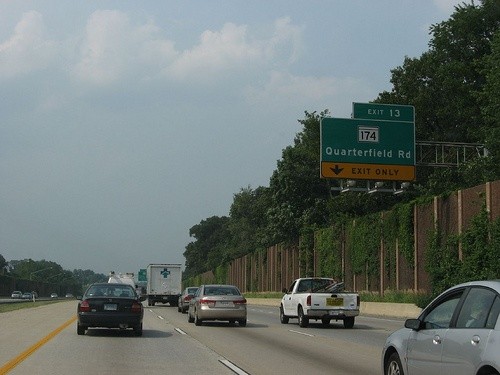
[280,276,361,328]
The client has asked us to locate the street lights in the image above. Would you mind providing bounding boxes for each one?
[30,267,53,280]
[47,272,66,282]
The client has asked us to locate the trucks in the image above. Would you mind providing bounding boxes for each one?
[145,264,183,307]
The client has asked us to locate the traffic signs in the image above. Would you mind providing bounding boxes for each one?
[319,116,417,183]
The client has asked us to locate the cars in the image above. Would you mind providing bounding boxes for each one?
[11,291,22,299]
[22,293,32,299]
[188,284,248,326]
[380,280,499,375]
[177,287,198,313]
[76,283,145,336]
[51,293,58,298]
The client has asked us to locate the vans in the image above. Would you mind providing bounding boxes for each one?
[108,275,137,292]
